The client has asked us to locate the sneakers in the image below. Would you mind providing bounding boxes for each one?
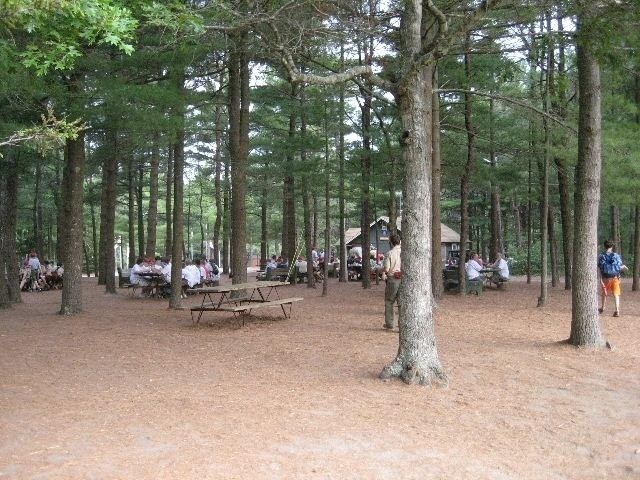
[612,310,620,317]
[383,324,395,329]
[598,308,604,314]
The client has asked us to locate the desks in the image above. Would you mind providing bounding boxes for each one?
[186,280,290,326]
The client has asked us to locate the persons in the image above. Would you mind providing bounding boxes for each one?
[130,253,221,299]
[17,251,65,292]
[596,238,629,318]
[465,252,486,294]
[381,234,403,330]
[487,252,510,290]
[255,245,385,283]
[472,254,484,269]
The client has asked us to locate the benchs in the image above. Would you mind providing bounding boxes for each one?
[188,297,306,328]
